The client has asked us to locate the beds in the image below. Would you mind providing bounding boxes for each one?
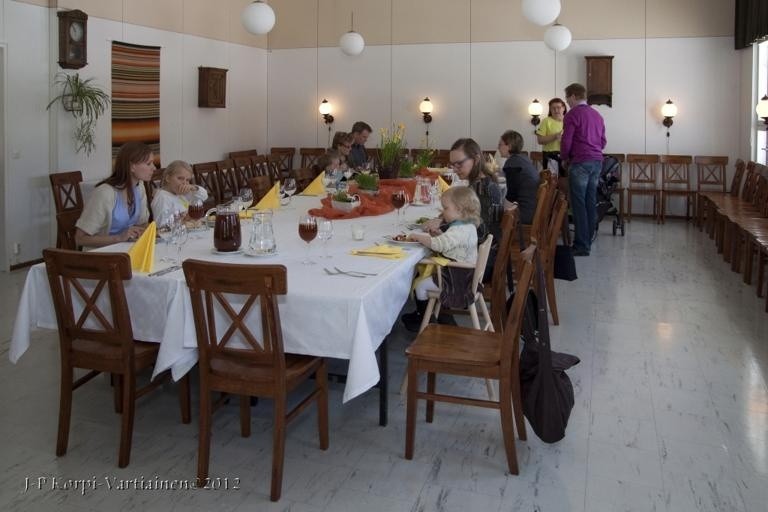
[8,179,470,427]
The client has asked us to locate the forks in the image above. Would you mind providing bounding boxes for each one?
[323,265,379,277]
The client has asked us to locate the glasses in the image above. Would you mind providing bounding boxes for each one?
[342,143,350,149]
[448,158,468,169]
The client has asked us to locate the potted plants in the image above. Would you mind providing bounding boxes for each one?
[331,189,361,212]
[354,173,380,196]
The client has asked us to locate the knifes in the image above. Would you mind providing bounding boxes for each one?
[333,264,368,282]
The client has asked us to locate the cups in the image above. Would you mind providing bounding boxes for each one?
[284,176,296,203]
[171,224,189,258]
[390,189,404,221]
[157,212,175,252]
[299,216,317,264]
[321,174,330,194]
[350,225,364,240]
[189,198,203,229]
[316,218,335,259]
[442,172,453,185]
[343,167,352,187]
[240,187,254,214]
[399,193,410,219]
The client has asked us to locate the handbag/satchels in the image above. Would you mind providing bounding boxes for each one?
[519,251,581,444]
[548,191,578,282]
[505,225,539,335]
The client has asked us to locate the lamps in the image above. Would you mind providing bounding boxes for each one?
[239,0,276,36]
[543,18,572,52]
[661,98,678,128]
[755,94,768,125]
[339,11,365,57]
[520,0,561,26]
[527,98,542,127]
[318,98,334,124]
[418,97,433,123]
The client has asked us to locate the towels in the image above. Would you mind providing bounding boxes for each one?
[353,245,401,259]
[411,257,449,289]
[126,221,156,272]
[302,171,326,195]
[437,175,448,192]
[256,180,280,208]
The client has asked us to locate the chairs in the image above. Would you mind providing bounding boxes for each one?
[482,150,497,163]
[404,236,538,476]
[703,158,768,312]
[143,167,166,224]
[694,155,729,226]
[411,149,427,166]
[366,147,381,171]
[216,158,239,202]
[299,147,326,169]
[626,153,662,224]
[181,257,330,501]
[270,147,295,177]
[399,233,496,402]
[265,152,282,186]
[511,191,567,326]
[529,179,550,296]
[530,151,544,170]
[660,155,697,224]
[248,153,267,178]
[233,156,252,193]
[48,170,85,252]
[41,246,192,469]
[192,162,230,216]
[439,201,518,334]
[603,153,625,221]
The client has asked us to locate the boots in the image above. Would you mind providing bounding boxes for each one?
[405,299,429,332]
[401,296,418,323]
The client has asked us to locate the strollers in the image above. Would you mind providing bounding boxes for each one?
[590,156,625,241]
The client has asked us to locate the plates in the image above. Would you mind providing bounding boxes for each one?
[392,237,420,246]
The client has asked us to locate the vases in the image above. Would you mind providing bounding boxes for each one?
[378,167,400,179]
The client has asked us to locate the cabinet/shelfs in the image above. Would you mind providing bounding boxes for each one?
[198,66,229,108]
[584,55,615,108]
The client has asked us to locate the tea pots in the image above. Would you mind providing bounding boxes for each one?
[250,209,277,255]
[414,173,433,204]
[206,204,247,252]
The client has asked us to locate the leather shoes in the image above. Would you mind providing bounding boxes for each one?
[570,245,589,256]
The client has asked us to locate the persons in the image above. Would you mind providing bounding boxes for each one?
[152,161,209,228]
[347,121,373,169]
[74,141,156,248]
[326,132,354,172]
[450,138,503,242]
[537,98,567,179]
[560,83,607,256]
[402,186,481,332]
[498,130,541,224]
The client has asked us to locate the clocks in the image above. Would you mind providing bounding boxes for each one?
[57,8,88,70]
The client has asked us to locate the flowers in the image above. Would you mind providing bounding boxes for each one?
[376,119,405,168]
